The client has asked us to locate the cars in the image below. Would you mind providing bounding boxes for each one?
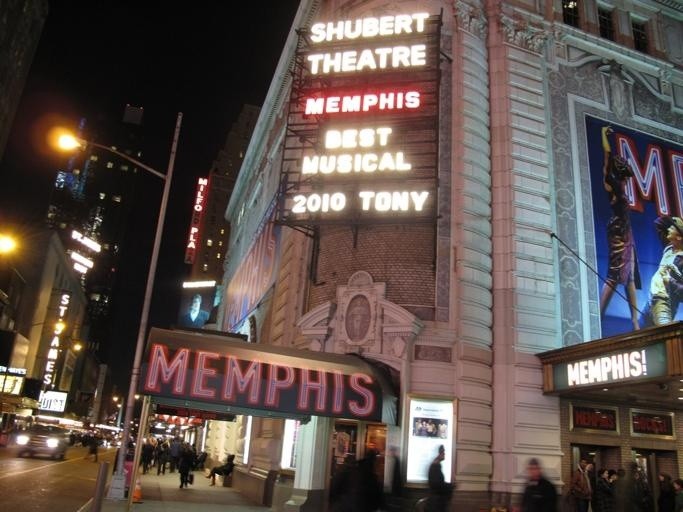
[15,424,71,462]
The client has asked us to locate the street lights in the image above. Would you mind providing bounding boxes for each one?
[44,106,188,501]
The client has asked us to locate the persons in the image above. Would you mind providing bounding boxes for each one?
[414,417,448,439]
[641,211,683,329]
[140,432,208,488]
[633,465,683,512]
[333,450,382,511]
[386,445,406,499]
[205,454,237,486]
[72,424,103,463]
[598,126,641,332]
[427,444,455,507]
[521,459,557,512]
[177,294,210,330]
[574,457,618,512]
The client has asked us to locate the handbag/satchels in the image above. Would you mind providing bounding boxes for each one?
[188,473,194,484]
